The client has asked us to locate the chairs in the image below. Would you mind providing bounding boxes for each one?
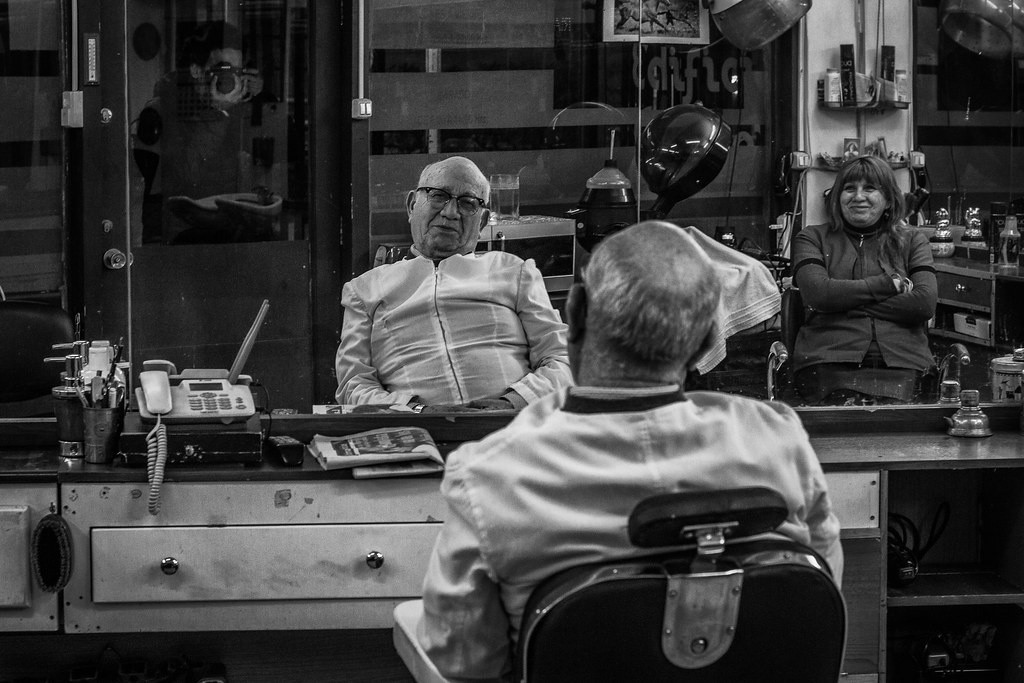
[764,285,971,404]
[398,485,848,683]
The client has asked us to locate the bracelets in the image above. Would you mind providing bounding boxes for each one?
[889,272,907,293]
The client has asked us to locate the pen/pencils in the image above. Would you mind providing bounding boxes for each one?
[74,344,124,407]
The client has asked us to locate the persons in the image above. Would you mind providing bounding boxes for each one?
[788,152,942,405]
[130,17,294,246]
[413,219,844,683]
[335,153,572,416]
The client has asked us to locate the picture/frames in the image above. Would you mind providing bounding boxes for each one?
[603,1,710,46]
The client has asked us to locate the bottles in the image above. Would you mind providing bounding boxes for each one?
[998,203,1020,267]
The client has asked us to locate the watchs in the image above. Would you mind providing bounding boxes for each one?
[412,403,430,414]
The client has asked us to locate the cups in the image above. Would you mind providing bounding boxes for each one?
[83,406,120,464]
[490,173,521,225]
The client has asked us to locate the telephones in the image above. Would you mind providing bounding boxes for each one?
[143,300,269,384]
[135,305,269,424]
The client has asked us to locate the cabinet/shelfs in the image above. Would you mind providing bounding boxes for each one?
[931,255,1024,349]
[475,214,577,301]
[875,460,1024,683]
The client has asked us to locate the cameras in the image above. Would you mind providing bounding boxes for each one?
[204,61,246,94]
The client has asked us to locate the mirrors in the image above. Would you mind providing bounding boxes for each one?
[0,0,1024,436]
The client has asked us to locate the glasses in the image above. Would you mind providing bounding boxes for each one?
[416,186,486,216]
